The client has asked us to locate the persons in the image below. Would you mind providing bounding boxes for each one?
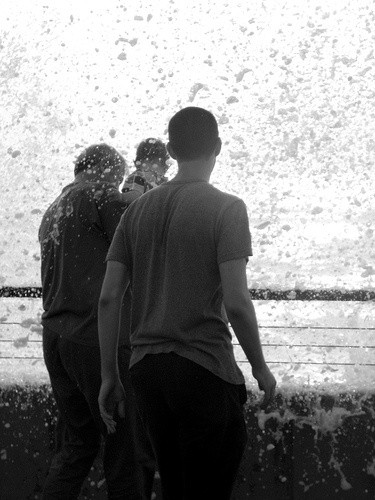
[97,106,277,500]
[121,137,171,195]
[37,142,134,500]
